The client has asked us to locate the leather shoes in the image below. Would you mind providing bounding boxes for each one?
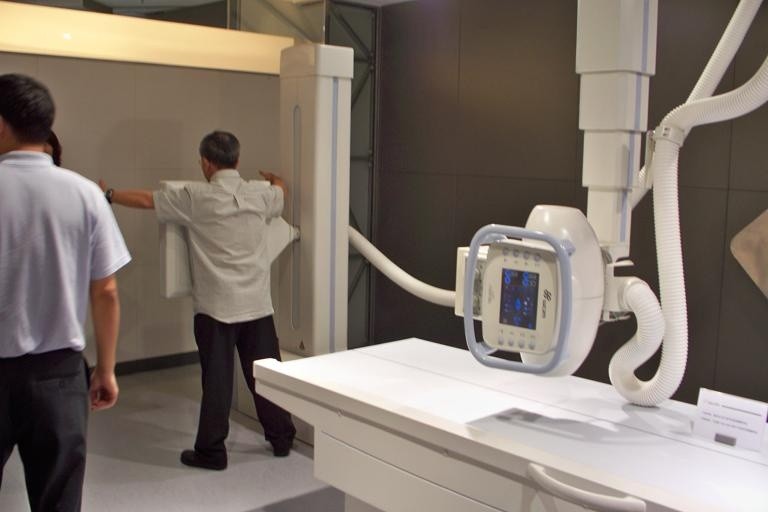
[180,450,227,470]
[274,446,290,456]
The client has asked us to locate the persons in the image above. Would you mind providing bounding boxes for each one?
[44,128,62,167]
[97,129,298,471]
[0,71,133,512]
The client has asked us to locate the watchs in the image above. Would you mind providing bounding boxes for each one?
[106,187,113,204]
